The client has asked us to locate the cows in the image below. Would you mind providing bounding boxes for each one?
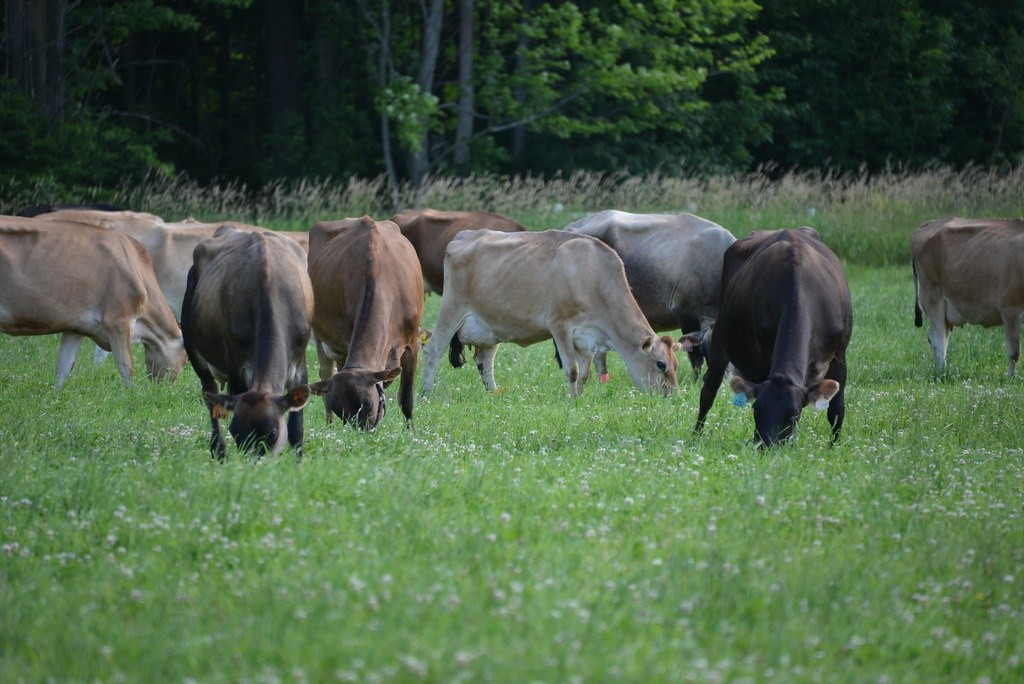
[0,210,854,470]
[912,216,1024,383]
